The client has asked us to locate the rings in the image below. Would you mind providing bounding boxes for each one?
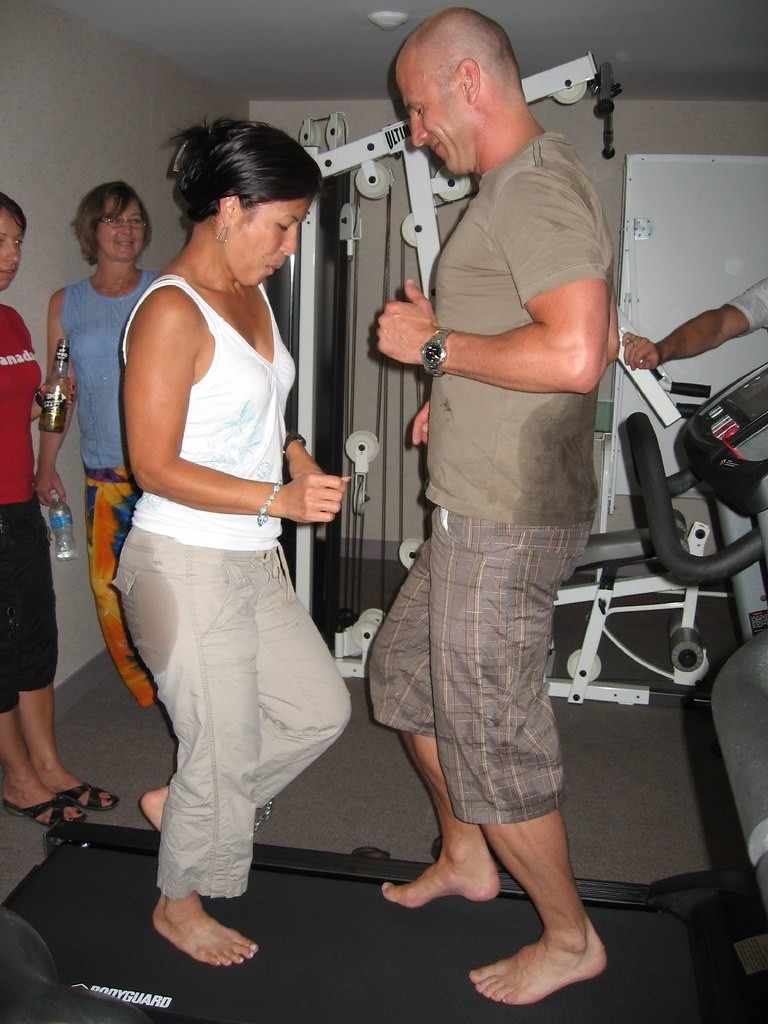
[630,340,635,346]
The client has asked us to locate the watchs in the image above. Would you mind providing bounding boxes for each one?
[419,328,452,376]
[284,432,306,463]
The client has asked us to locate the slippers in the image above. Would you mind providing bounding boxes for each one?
[54,781,120,812]
[3,792,89,827]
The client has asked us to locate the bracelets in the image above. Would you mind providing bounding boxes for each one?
[35,388,44,407]
[257,483,281,526]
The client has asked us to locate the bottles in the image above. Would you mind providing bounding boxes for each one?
[48,493,78,560]
[39,339,70,433]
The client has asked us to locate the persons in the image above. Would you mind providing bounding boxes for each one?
[367,6,619,1005]
[111,115,352,966]
[33,180,162,706]
[622,277,768,371]
[0,191,120,826]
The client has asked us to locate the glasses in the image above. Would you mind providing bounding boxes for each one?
[99,218,147,229]
